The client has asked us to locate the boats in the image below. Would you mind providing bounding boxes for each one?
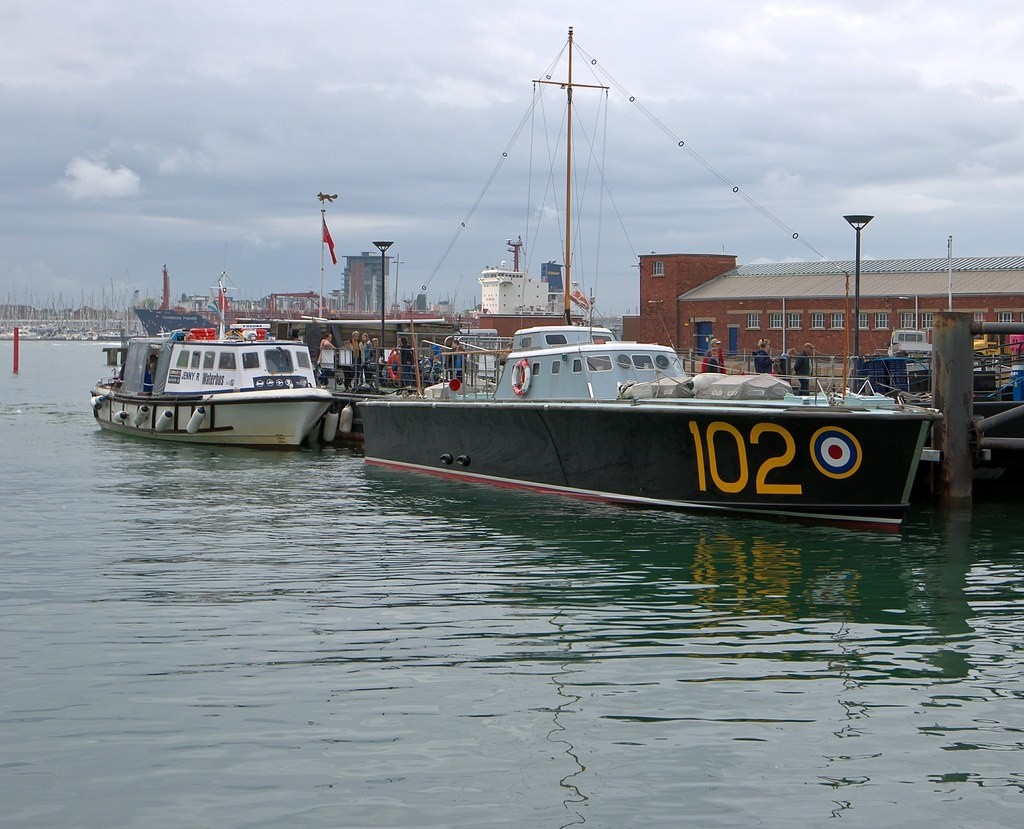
[89,271,334,451]
[356,26,945,536]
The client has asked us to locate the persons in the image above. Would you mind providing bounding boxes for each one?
[317,331,467,389]
[145,354,159,384]
[120,361,126,381]
[507,343,512,354]
[701,339,815,396]
[291,328,302,341]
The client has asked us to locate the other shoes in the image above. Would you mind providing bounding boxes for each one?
[349,384,352,388]
[344,382,349,389]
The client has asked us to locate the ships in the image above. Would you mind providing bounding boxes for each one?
[131,265,442,338]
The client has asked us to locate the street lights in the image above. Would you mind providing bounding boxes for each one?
[842,214,874,393]
[372,241,394,357]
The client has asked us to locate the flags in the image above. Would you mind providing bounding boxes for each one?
[322,212,338,265]
[208,288,222,317]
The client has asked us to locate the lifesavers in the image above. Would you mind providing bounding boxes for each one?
[388,355,402,379]
[510,359,531,396]
[190,327,216,340]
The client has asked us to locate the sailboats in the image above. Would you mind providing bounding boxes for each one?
[0,279,150,341]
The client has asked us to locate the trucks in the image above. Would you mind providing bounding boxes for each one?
[885,328,932,358]
[974,332,1024,359]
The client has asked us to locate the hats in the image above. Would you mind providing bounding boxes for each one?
[804,343,816,348]
[711,339,721,343]
